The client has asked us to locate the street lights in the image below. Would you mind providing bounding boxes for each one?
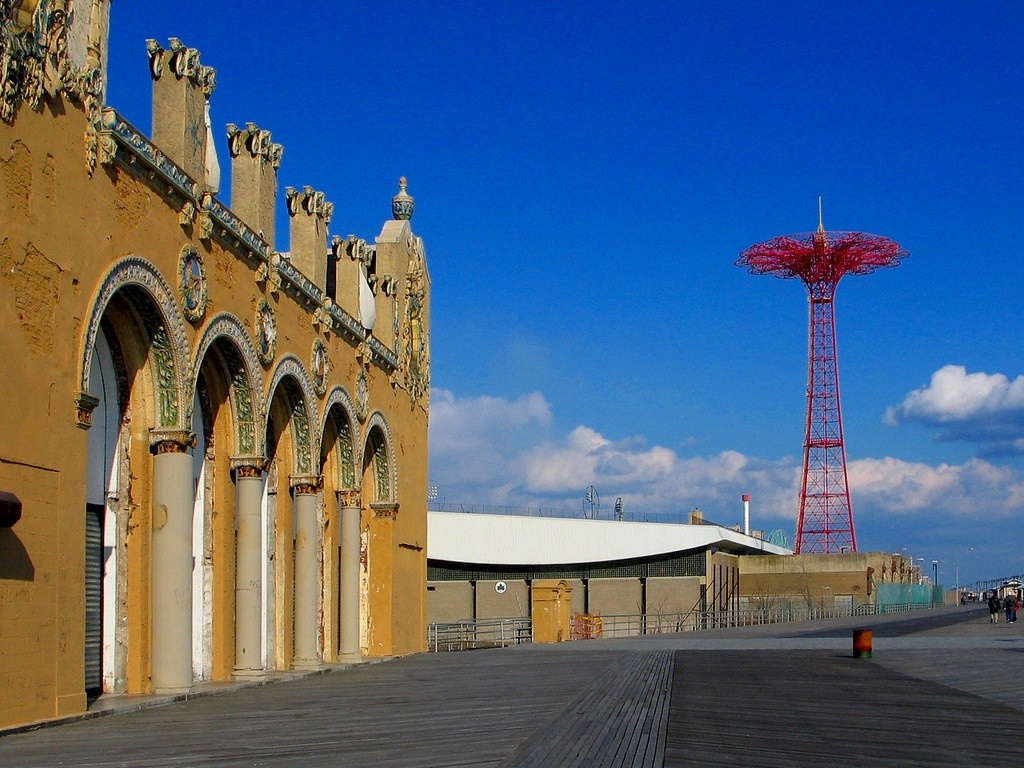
[955,547,975,607]
[585,485,596,520]
[910,558,924,583]
[615,498,624,521]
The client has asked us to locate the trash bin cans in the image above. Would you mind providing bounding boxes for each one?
[853,630,872,658]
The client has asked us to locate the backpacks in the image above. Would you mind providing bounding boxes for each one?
[1006,596,1013,607]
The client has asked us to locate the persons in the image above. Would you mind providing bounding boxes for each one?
[1003,591,1020,624]
[988,593,1001,623]
[962,595,967,605]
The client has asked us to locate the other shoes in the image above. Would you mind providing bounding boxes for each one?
[991,619,993,623]
[1010,621,1013,624]
